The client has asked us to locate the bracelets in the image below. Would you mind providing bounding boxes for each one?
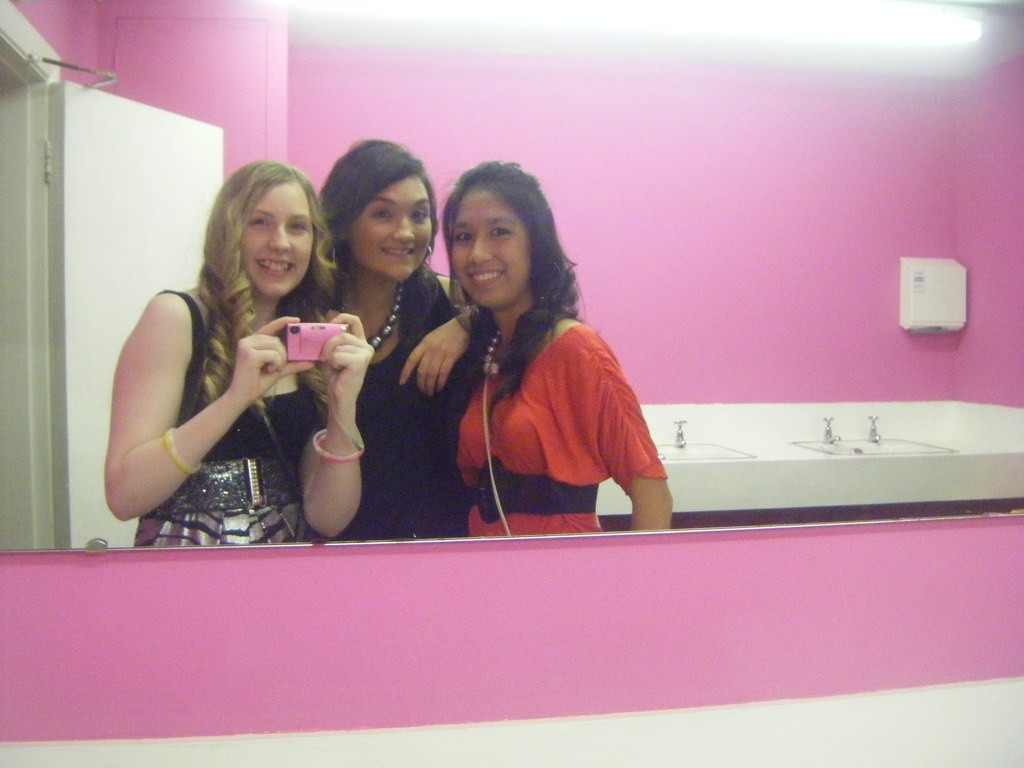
[312,429,365,464]
[163,427,202,476]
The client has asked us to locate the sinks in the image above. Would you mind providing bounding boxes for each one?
[655,441,759,468]
[789,437,960,461]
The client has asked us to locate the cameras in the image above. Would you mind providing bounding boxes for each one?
[285,323,348,361]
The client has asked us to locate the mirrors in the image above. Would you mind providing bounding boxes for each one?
[0,0,1024,553]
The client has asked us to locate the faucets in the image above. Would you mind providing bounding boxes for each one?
[673,419,688,448]
[868,415,881,443]
[823,416,842,444]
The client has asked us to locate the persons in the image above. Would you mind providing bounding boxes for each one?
[106,140,673,546]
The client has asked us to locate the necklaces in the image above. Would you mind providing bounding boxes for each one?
[483,329,500,379]
[367,280,403,351]
